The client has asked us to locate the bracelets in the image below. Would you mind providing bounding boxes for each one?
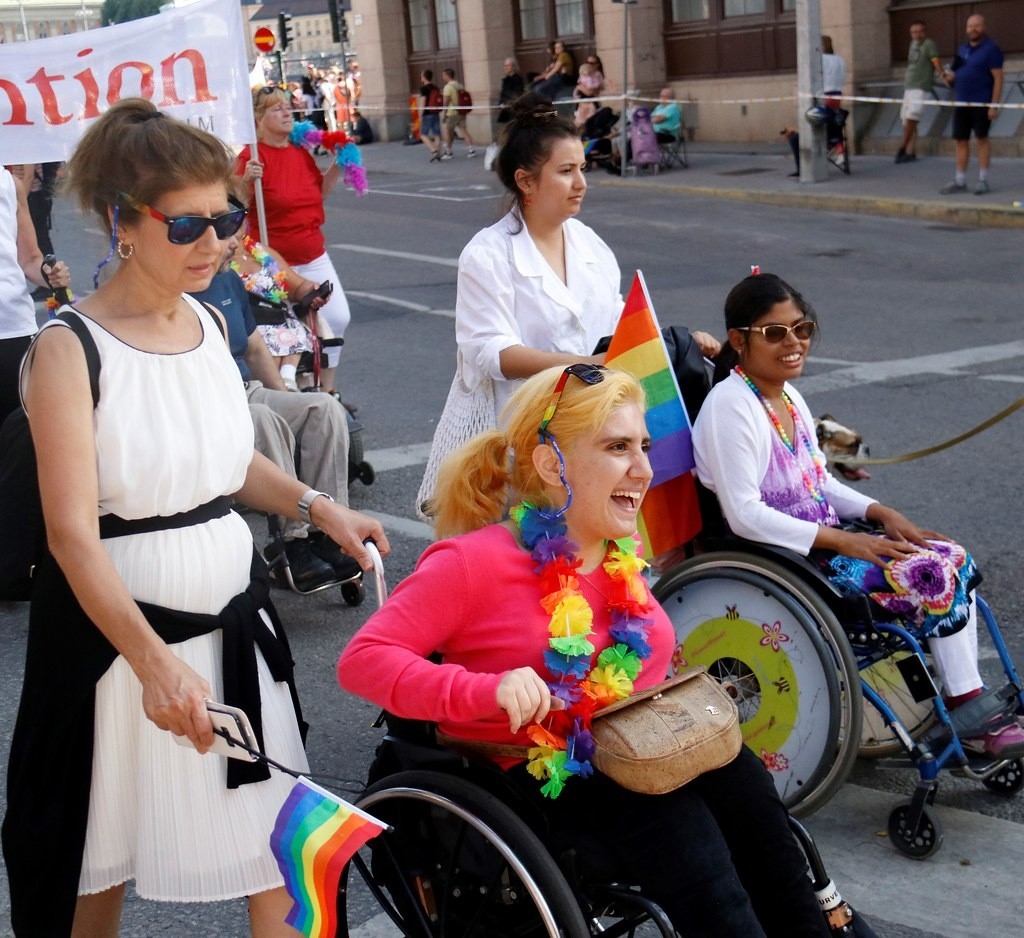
[298,489,334,530]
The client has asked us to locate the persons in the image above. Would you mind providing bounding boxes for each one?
[0,40,683,610]
[692,271,1024,755]
[336,361,836,938]
[939,13,1004,194]
[415,94,722,531]
[1,98,391,938]
[895,20,947,162]
[784,37,845,177]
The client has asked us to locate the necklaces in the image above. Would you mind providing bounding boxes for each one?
[506,498,652,803]
[733,365,827,503]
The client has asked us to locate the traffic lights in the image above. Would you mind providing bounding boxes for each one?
[328,0,348,43]
[279,12,293,51]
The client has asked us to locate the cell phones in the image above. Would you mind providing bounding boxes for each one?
[170,698,260,764]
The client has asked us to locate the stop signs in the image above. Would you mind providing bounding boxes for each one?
[254,28,275,53]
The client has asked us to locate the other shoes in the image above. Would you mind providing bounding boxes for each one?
[429,150,441,162]
[29,286,54,302]
[441,151,454,159]
[948,700,1024,758]
[940,180,968,194]
[894,147,916,164]
[787,171,799,177]
[467,149,477,158]
[277,541,334,591]
[974,180,989,195]
[309,531,360,576]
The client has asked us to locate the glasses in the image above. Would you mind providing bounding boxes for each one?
[739,319,817,344]
[540,363,610,445]
[256,86,285,108]
[118,190,249,245]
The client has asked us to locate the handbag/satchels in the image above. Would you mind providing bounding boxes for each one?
[586,662,741,792]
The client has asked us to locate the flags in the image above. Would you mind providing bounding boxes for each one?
[602,269,696,492]
[270,775,389,938]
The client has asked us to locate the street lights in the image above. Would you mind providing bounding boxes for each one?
[611,0,639,178]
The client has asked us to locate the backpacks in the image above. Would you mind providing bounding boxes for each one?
[0,309,102,606]
[423,86,444,113]
[448,84,472,114]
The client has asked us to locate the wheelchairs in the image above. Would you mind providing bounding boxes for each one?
[204,532,846,938]
[245,279,376,608]
[648,350,1024,862]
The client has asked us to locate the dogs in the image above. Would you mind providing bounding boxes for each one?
[813,413,873,481]
